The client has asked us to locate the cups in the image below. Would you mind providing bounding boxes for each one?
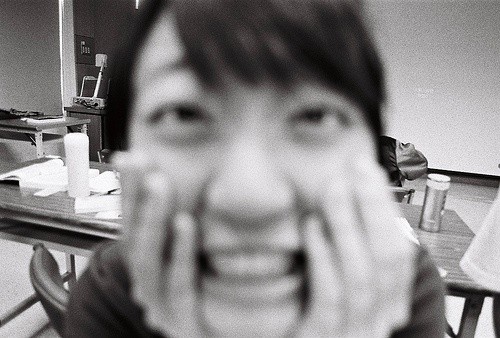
[63,133,90,198]
[419,173,451,232]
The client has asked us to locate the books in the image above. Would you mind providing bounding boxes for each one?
[0,157,124,217]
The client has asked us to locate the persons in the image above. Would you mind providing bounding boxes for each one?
[64,1,448,338]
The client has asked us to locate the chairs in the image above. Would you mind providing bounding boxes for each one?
[28,242,73,338]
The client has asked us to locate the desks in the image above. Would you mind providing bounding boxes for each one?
[0,155,500,338]
[0,106,105,160]
